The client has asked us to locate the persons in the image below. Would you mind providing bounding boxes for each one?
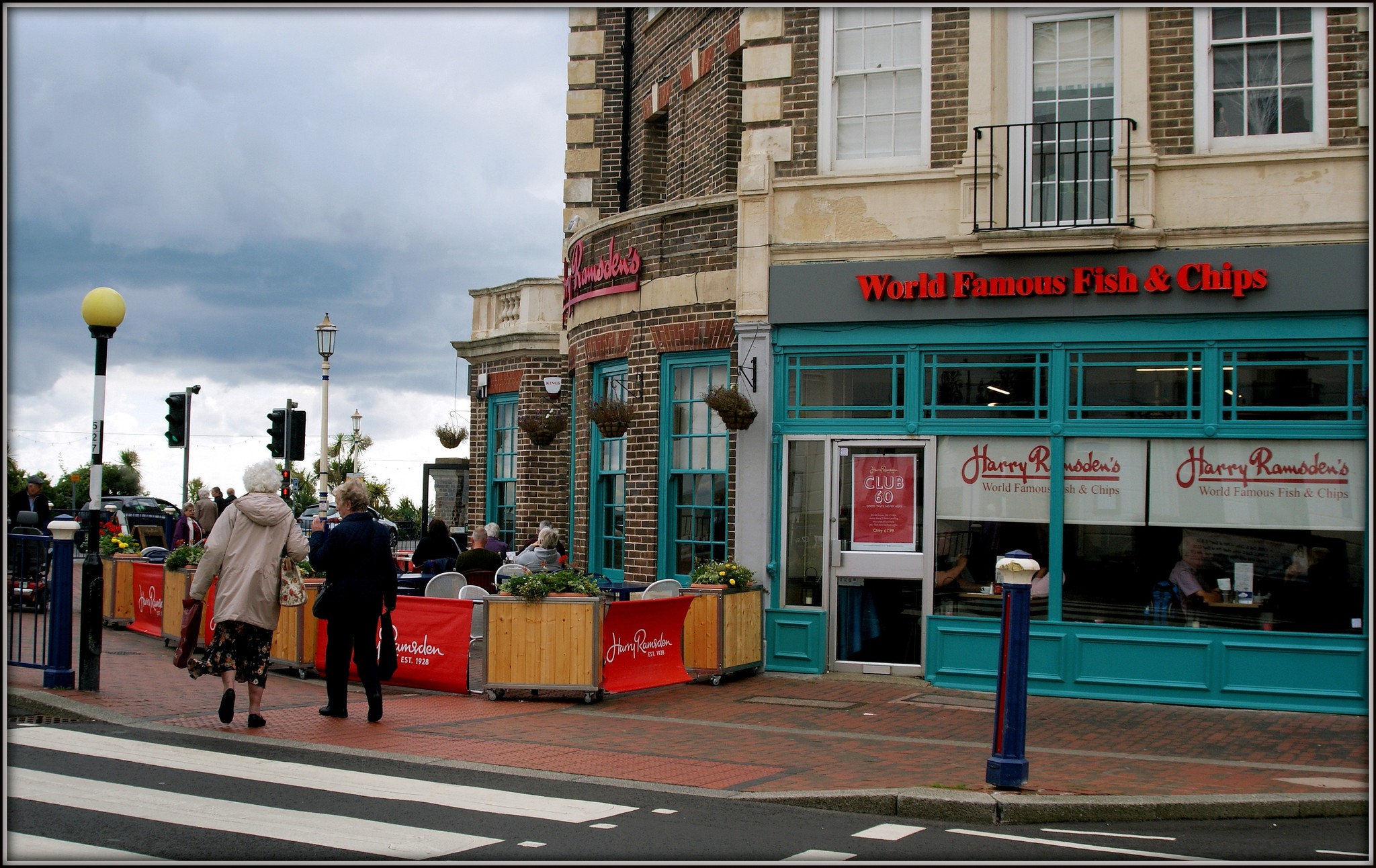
[193,487,219,539]
[224,488,237,506]
[411,518,566,574]
[934,519,1319,631]
[188,459,311,727]
[308,477,398,722]
[169,501,205,552]
[8,474,49,581]
[211,487,226,519]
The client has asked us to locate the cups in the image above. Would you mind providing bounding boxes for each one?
[981,586,990,591]
[513,556,517,564]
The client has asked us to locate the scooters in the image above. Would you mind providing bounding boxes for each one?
[7,511,53,614]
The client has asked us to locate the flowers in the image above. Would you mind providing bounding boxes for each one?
[164,538,208,571]
[295,559,326,579]
[80,521,144,558]
[688,556,757,588]
[491,555,612,605]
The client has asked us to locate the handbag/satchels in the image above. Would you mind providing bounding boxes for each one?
[311,583,335,620]
[279,555,309,607]
[379,609,398,681]
[172,598,204,669]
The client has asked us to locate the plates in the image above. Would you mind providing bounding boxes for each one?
[980,591,990,594]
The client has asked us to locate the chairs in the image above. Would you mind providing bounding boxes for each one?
[417,564,682,602]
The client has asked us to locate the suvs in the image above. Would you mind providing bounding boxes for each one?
[74,496,183,535]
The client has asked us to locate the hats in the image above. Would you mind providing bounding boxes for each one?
[27,475,46,485]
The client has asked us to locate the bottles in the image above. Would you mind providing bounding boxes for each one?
[498,552,501,558]
[994,571,1002,595]
[503,560,505,565]
[989,582,994,595]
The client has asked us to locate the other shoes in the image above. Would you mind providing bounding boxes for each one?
[319,705,348,719]
[218,688,237,724]
[367,690,383,723]
[247,712,267,729]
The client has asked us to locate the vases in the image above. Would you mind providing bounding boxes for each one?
[690,583,752,589]
[111,554,139,557]
[499,592,591,596]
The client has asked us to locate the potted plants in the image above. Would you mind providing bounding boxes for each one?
[585,395,634,438]
[700,384,758,430]
[434,423,470,449]
[516,414,568,446]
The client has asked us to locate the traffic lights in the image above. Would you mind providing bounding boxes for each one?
[164,392,187,448]
[280,470,291,485]
[281,487,290,503]
[266,408,287,459]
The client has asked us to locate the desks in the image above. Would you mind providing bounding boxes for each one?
[1208,588,1259,608]
[594,583,650,601]
[392,552,413,571]
[959,593,1038,600]
[397,573,436,596]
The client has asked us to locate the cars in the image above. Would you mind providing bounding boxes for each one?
[297,502,398,547]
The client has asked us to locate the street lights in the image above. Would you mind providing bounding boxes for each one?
[350,409,363,473]
[79,286,126,692]
[314,313,339,533]
